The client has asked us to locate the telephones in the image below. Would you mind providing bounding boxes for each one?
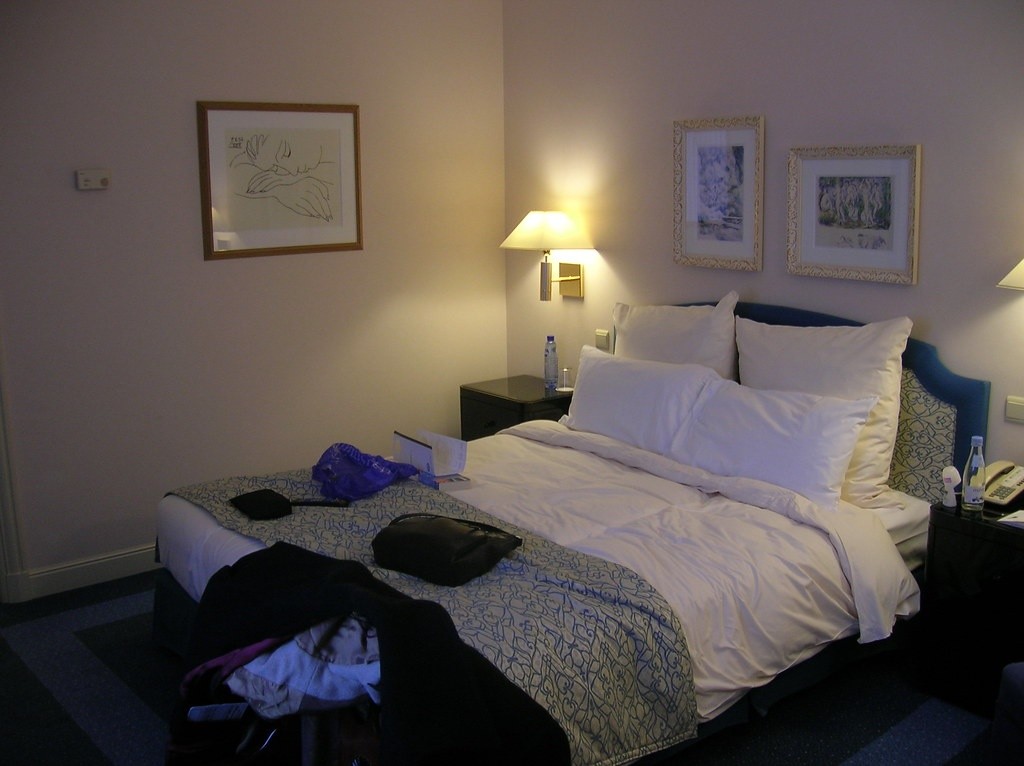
[970,460,1024,509]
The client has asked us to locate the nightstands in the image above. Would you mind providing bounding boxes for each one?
[460,374,572,440]
[925,501,1024,704]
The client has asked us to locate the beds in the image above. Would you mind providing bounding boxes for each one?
[156,300,991,766]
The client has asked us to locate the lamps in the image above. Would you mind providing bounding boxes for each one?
[996,256,1024,293]
[499,211,596,300]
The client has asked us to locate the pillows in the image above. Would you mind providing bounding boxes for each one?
[668,377,881,509]
[614,290,738,380]
[735,315,912,512]
[559,345,723,455]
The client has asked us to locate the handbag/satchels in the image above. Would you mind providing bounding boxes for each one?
[372,513,522,587]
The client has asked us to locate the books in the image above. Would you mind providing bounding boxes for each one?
[418,470,472,491]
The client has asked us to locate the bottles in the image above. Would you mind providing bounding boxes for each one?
[962,436,986,511]
[544,335,558,390]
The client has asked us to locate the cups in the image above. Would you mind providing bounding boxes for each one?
[558,368,574,390]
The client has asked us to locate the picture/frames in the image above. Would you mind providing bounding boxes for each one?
[672,115,765,273]
[196,100,364,261]
[785,143,922,285]
